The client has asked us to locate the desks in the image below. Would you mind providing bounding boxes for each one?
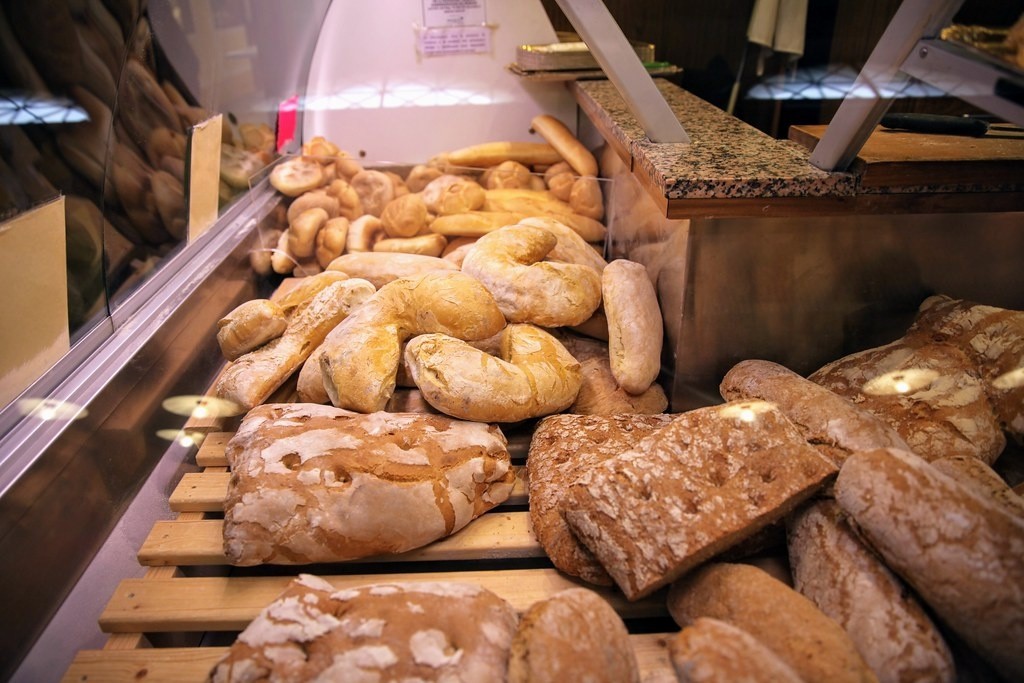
[748,62,952,140]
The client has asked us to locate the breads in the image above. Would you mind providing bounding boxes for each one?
[218,114,1024,683]
[0,0,274,321]
